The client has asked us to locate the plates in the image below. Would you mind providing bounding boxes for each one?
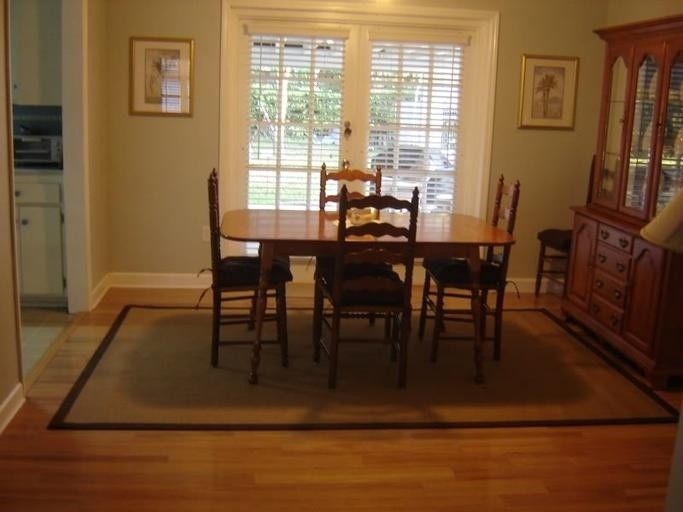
[332,218,381,228]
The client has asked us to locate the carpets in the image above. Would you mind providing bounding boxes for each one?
[44,302,683,431]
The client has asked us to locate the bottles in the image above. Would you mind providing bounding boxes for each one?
[640,67,683,159]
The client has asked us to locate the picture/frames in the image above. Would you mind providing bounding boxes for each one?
[126,34,194,119]
[516,53,580,132]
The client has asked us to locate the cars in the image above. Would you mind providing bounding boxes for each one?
[370,147,453,203]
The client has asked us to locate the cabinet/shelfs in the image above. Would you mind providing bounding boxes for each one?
[13,183,65,309]
[10,0,61,107]
[559,13,682,391]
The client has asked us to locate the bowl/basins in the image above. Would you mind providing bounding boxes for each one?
[346,206,376,226]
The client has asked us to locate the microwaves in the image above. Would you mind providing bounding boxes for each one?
[12,133,61,166]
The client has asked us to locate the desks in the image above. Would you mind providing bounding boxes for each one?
[218,207,517,384]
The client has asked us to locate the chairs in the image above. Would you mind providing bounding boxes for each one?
[205,167,295,367]
[417,173,522,364]
[313,162,395,282]
[311,185,419,389]
[534,152,594,299]
